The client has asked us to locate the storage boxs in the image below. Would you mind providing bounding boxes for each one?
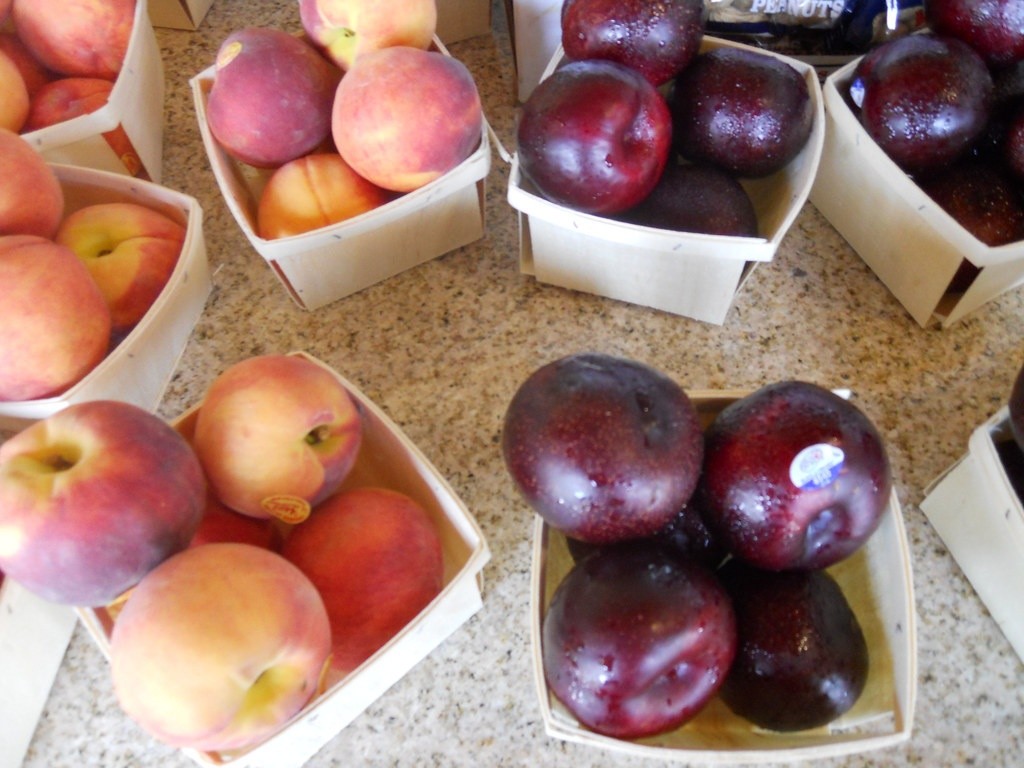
[189,32,512,311]
[72,350,492,768]
[0,0,165,186]
[507,36,826,328]
[918,405,1024,663]
[0,163,216,442]
[808,29,1024,331]
[529,392,918,764]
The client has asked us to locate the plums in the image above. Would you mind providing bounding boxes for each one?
[847,0,1024,248]
[513,0,812,238]
[500,353,891,738]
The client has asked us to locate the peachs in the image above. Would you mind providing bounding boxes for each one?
[0,356,450,759]
[204,0,484,240]
[0,0,135,137]
[0,128,187,405]
